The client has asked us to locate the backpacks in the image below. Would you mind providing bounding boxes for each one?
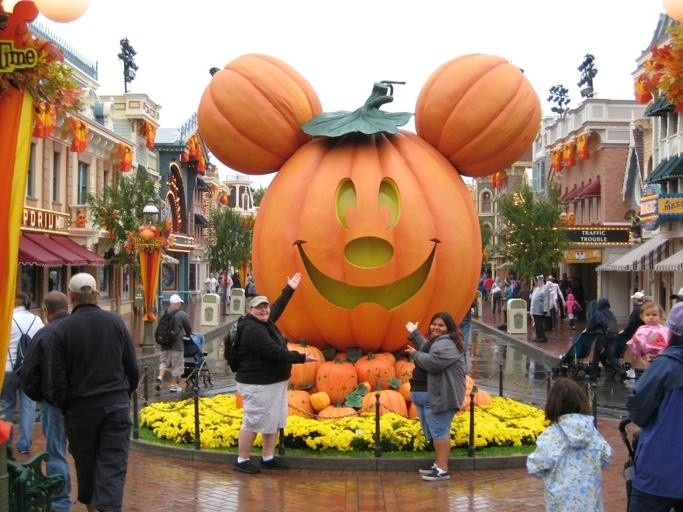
[154,307,179,345]
[7,315,36,371]
[223,316,249,372]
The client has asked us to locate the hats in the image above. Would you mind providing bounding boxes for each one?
[630,292,644,300]
[665,287,683,337]
[66,272,96,294]
[248,295,269,307]
[169,294,184,304]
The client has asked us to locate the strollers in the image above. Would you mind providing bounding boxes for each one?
[599,327,630,379]
[558,324,596,379]
[181,331,216,394]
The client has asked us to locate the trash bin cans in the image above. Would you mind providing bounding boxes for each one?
[200,293,220,327]
[477,290,482,317]
[230,288,245,315]
[507,297,527,336]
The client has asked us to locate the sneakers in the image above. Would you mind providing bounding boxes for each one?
[531,336,546,342]
[233,455,258,474]
[169,385,181,392]
[154,378,161,391]
[418,462,450,480]
[259,455,288,470]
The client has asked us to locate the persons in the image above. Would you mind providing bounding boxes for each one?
[47,271,142,511]
[17,290,74,511]
[525,379,612,511]
[402,310,466,481]
[626,301,682,512]
[153,293,193,393]
[204,265,255,306]
[223,271,319,475]
[475,274,682,384]
[0,290,45,457]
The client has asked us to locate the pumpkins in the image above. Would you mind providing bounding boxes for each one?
[197,52,323,174]
[414,54,542,178]
[285,337,492,421]
[252,80,483,353]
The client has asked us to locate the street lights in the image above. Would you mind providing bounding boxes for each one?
[118,35,143,96]
[548,82,573,122]
[576,51,600,103]
[140,195,162,352]
